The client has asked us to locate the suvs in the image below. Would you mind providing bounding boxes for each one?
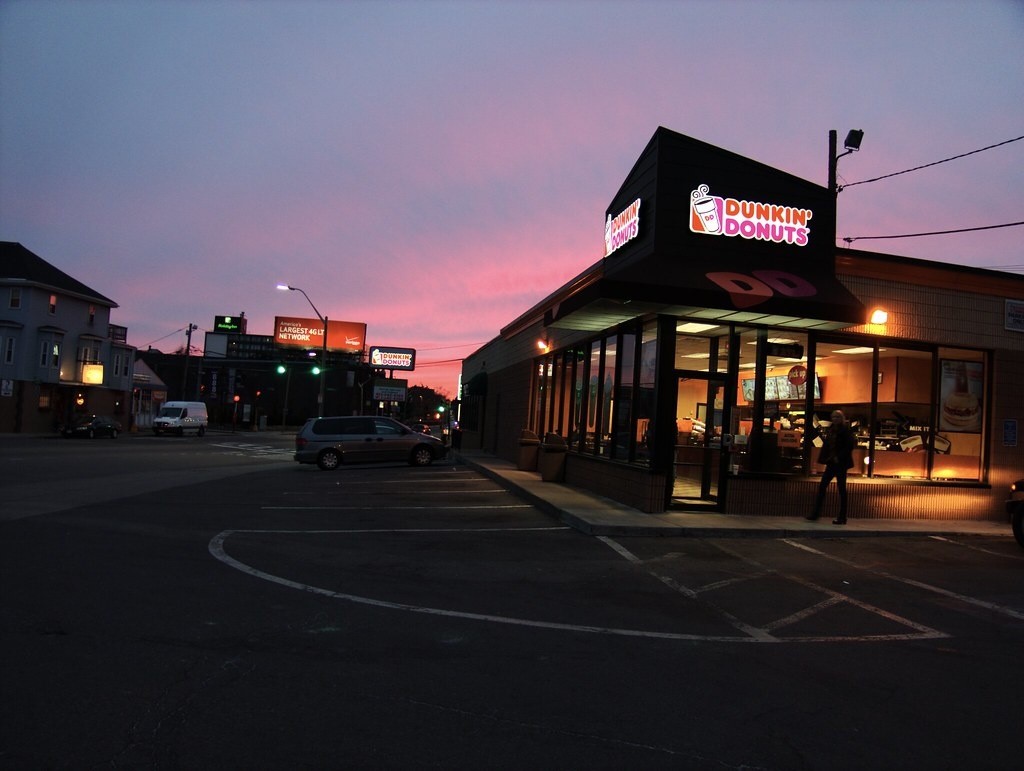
[293,414,449,472]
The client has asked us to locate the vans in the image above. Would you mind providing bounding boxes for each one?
[152,401,209,437]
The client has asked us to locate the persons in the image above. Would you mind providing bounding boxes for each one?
[807,409,854,524]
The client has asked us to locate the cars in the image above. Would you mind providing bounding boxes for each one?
[410,424,432,435]
[61,415,124,439]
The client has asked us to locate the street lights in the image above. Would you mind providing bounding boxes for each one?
[287,284,328,417]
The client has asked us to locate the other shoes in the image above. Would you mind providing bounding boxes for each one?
[807,514,820,520]
[832,517,847,524]
